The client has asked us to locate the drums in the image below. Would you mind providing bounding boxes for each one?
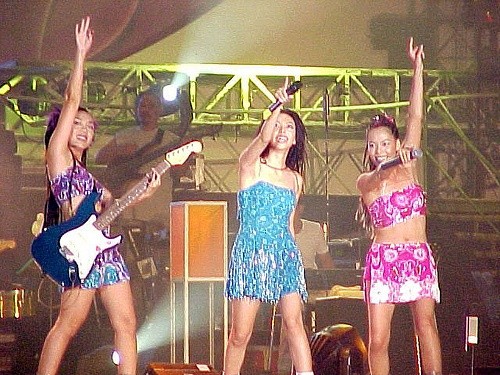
[0,290,38,322]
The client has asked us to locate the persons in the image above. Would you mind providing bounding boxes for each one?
[352,6,448,111]
[355,35,444,375]
[95,91,194,318]
[143,62,192,117]
[270,195,341,374]
[36,15,162,375]
[222,87,316,375]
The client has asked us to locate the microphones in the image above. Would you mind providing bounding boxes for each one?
[381,149,423,170]
[268,80,303,112]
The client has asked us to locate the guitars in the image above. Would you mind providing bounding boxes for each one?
[30,140,203,291]
[104,124,223,199]
[0,237,15,250]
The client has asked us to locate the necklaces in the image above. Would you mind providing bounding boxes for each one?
[261,155,287,171]
